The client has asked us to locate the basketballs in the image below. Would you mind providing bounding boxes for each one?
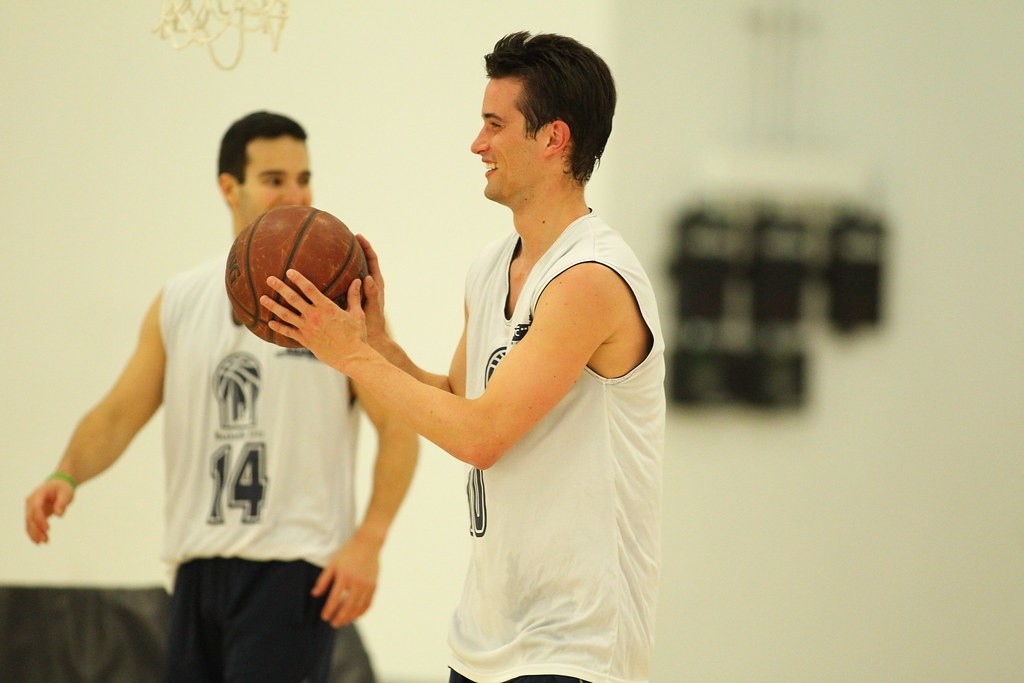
[224,208,369,349]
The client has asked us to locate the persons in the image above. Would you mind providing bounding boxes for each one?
[22,109,420,683]
[261,30,669,683]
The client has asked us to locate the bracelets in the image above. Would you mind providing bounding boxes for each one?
[48,472,79,488]
